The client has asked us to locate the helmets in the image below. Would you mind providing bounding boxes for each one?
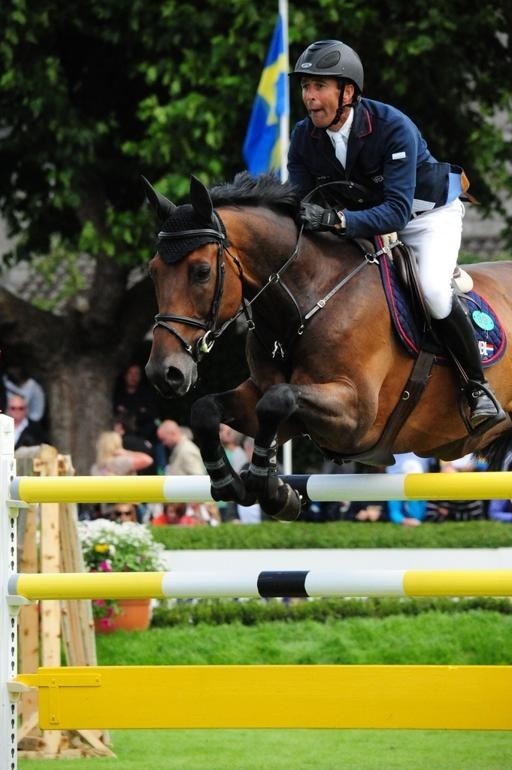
[288,39,364,93]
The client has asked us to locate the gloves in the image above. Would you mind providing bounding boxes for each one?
[299,201,342,233]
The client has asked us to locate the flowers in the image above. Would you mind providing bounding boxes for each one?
[36,518,179,628]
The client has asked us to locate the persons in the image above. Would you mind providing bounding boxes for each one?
[287,40,506,429]
[88,363,263,524]
[1,341,45,449]
[301,452,512,526]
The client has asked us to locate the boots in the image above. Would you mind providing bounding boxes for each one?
[432,292,511,438]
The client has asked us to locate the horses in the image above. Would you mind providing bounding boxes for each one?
[131,168,512,523]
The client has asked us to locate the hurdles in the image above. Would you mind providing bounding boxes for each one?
[0,411,512,770]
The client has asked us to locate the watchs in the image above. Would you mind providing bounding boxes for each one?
[338,205,347,233]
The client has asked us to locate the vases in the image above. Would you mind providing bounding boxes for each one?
[89,569,153,635]
